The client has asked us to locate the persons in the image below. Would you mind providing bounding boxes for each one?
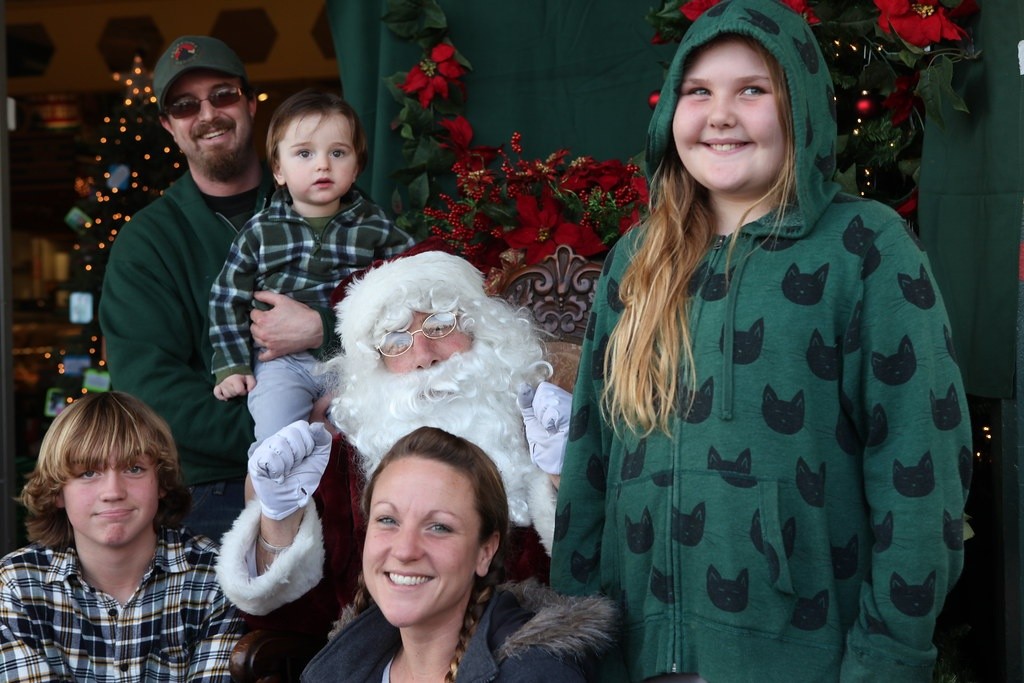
[549,0,973,683]
[213,238,578,636]
[98,34,342,535]
[0,389,257,683]
[209,89,412,447]
[301,427,617,683]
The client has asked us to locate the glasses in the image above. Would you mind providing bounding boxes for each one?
[164,84,248,119]
[376,311,460,356]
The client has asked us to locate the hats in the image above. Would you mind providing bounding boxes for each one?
[153,35,249,115]
[330,235,491,372]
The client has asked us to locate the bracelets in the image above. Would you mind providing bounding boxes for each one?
[257,535,288,553]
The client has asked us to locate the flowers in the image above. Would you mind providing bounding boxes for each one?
[378,1,980,279]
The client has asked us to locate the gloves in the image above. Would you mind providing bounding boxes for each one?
[516,380,572,474]
[248,419,332,520]
